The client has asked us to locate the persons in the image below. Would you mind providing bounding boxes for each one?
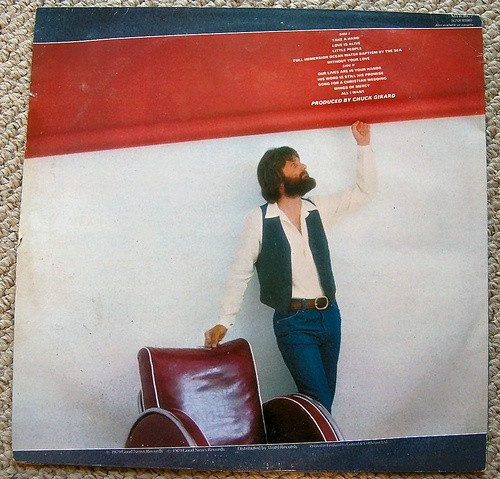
[204,121,380,417]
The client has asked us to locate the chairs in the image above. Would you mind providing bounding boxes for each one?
[124,338,344,448]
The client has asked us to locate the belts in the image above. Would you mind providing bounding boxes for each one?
[291,296,330,310]
